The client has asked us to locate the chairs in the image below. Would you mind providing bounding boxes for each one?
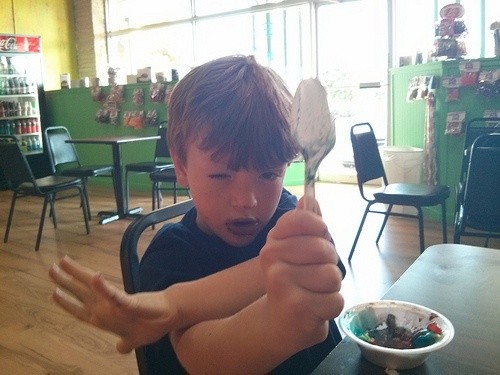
[452,117,500,250]
[0,136,91,251]
[45,126,114,220]
[348,122,450,260]
[125,121,184,214]
[150,168,198,230]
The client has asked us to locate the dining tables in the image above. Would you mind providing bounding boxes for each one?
[65,136,162,225]
[311,244,500,375]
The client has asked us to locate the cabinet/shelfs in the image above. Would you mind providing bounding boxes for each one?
[0,33,43,188]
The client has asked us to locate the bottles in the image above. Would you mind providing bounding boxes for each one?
[16,135,41,153]
[0,77,36,95]
[0,99,36,117]
[0,118,39,135]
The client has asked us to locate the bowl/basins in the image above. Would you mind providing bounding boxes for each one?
[339,300,455,369]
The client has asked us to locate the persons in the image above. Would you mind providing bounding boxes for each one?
[47,55,346,374]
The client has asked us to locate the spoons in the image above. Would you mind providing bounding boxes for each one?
[289,77,337,215]
